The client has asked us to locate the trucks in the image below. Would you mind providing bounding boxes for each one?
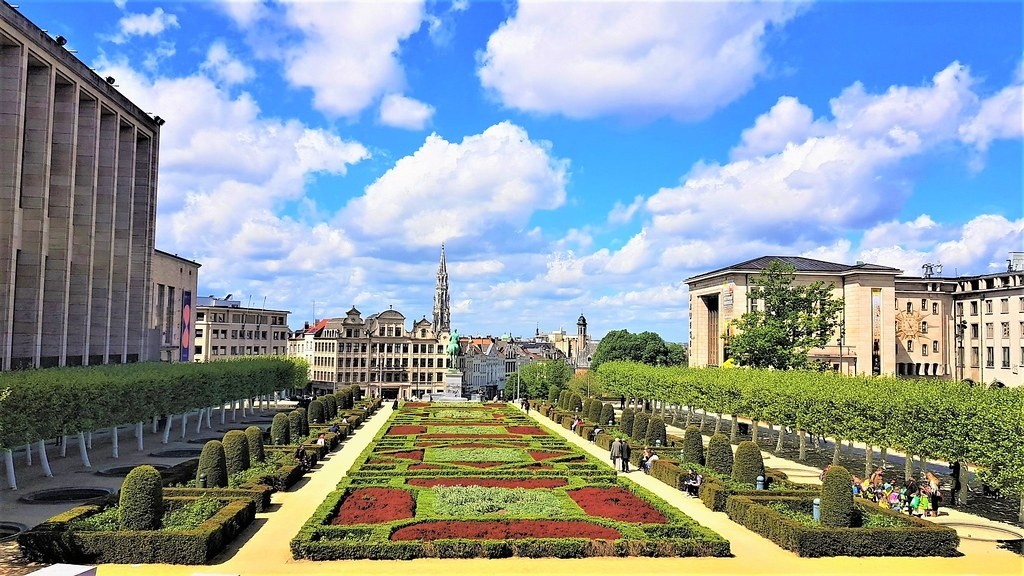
[317,389,323,397]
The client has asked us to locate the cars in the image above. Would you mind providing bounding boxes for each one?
[279,398,290,401]
[304,395,312,400]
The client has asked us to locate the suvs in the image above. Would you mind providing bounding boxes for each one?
[290,395,303,401]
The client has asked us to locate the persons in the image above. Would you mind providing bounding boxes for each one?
[638,446,659,473]
[316,435,324,447]
[295,444,306,461]
[685,469,703,498]
[341,416,347,424]
[392,398,398,410]
[525,401,530,414]
[449,329,460,344]
[949,459,961,503]
[572,415,583,431]
[851,465,942,518]
[329,423,339,433]
[610,437,631,473]
